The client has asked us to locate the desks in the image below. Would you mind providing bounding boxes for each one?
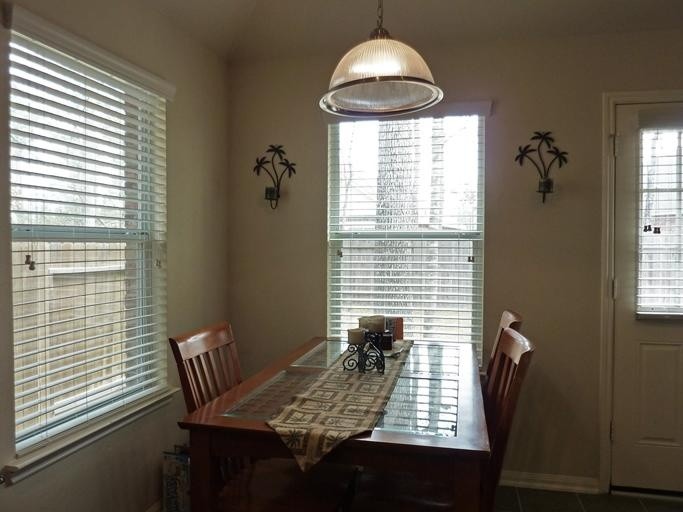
[176,336,493,510]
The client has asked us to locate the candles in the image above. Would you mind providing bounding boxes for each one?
[347,316,393,350]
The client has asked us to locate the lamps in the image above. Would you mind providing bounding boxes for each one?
[316,0,445,119]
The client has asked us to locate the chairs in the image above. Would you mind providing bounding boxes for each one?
[401,327,535,510]
[480,310,525,397]
[167,321,292,509]
